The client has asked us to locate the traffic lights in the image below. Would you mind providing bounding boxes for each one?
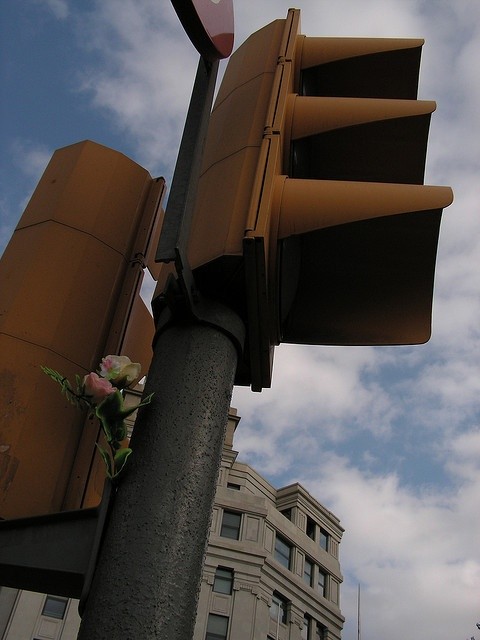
[185,7,454,392]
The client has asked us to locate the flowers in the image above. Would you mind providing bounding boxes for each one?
[39,355,155,486]
